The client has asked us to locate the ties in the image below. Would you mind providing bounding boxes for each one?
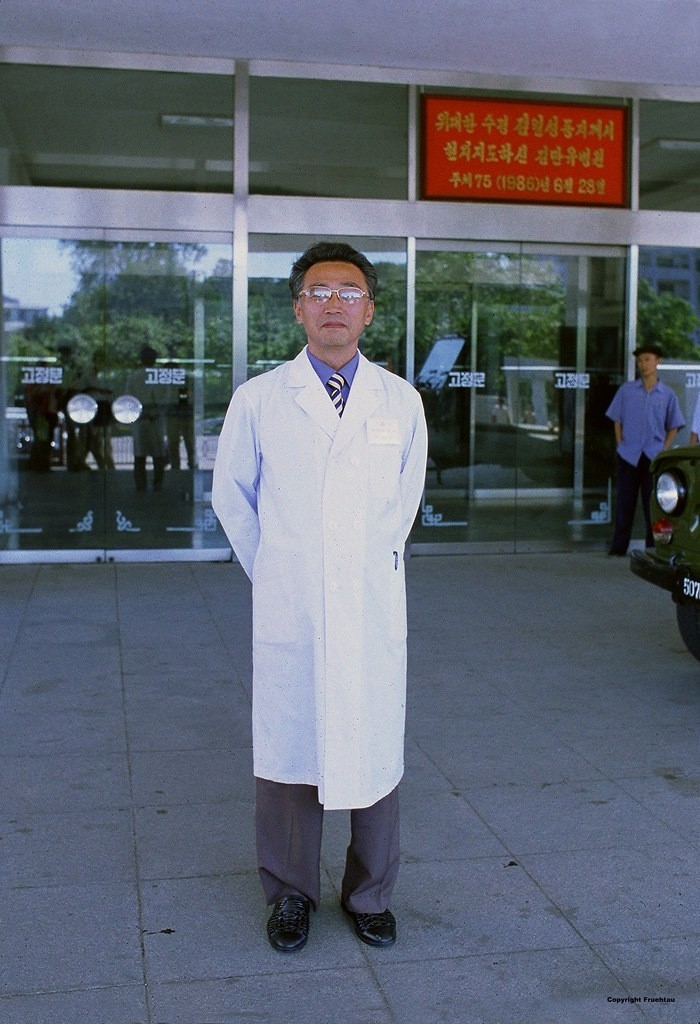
[326,373,345,419]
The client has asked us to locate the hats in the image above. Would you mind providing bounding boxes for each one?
[633,345,666,357]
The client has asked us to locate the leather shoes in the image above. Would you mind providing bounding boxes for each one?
[341,903,396,947]
[267,895,310,952]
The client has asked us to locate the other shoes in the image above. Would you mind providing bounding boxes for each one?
[137,480,149,492]
[607,547,626,559]
[153,481,162,491]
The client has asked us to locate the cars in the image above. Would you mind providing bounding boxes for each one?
[629,443,700,662]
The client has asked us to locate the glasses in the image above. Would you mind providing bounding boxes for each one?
[295,285,371,305]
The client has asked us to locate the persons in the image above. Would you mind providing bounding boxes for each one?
[689,389,700,446]
[491,397,513,425]
[211,244,430,953]
[23,343,198,492]
[605,345,686,556]
[522,406,535,424]
